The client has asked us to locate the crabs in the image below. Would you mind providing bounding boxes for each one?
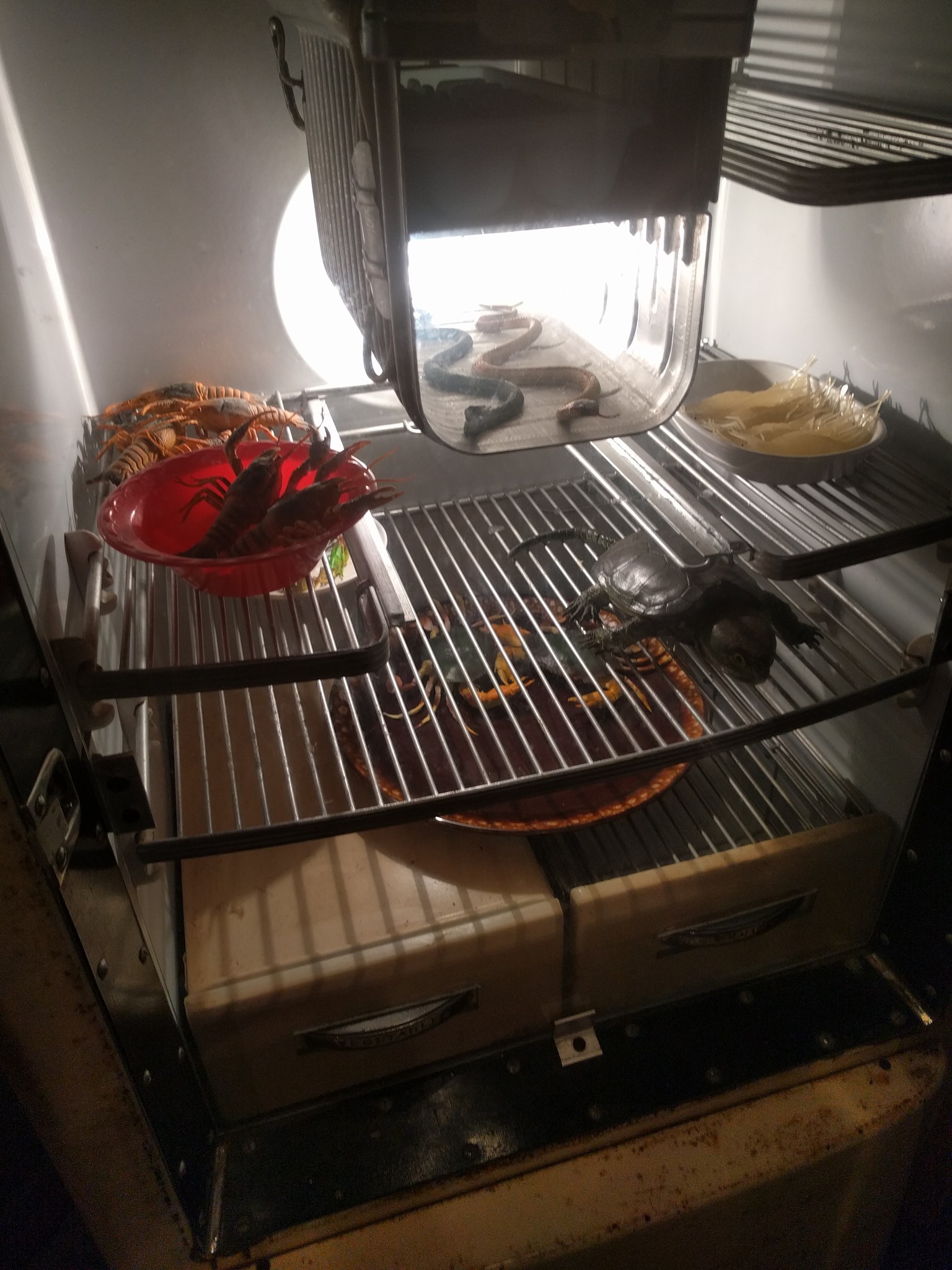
[378,593,673,736]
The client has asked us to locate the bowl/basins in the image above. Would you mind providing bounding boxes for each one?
[328,592,706,832]
[97,439,378,597]
[667,359,887,486]
[265,516,388,597]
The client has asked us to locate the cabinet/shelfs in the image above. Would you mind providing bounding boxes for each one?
[64,90,952,1119]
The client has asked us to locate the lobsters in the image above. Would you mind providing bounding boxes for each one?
[86,381,404,558]
